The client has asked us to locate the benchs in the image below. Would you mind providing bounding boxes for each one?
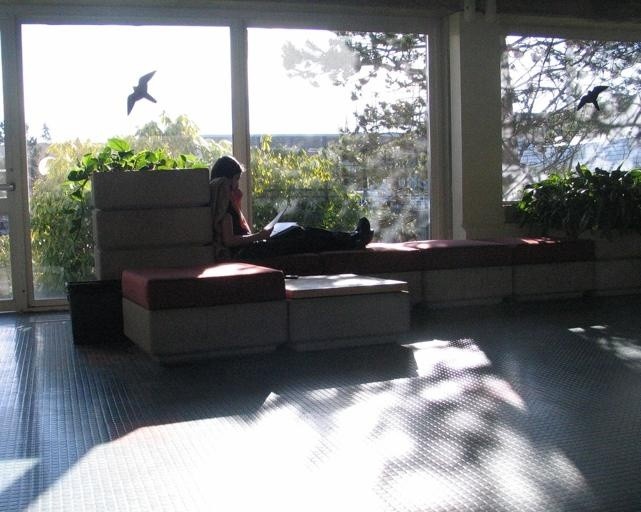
[224,234,595,309]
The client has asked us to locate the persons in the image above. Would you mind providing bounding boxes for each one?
[210,155,374,260]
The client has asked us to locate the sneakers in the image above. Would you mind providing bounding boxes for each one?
[356,217,371,237]
[356,229,374,248]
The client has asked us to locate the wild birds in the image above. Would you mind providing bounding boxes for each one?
[127,70,157,115]
[575,84,611,113]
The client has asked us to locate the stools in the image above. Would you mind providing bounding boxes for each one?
[121,262,412,366]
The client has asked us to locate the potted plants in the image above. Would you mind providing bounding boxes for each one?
[509,160,641,297]
